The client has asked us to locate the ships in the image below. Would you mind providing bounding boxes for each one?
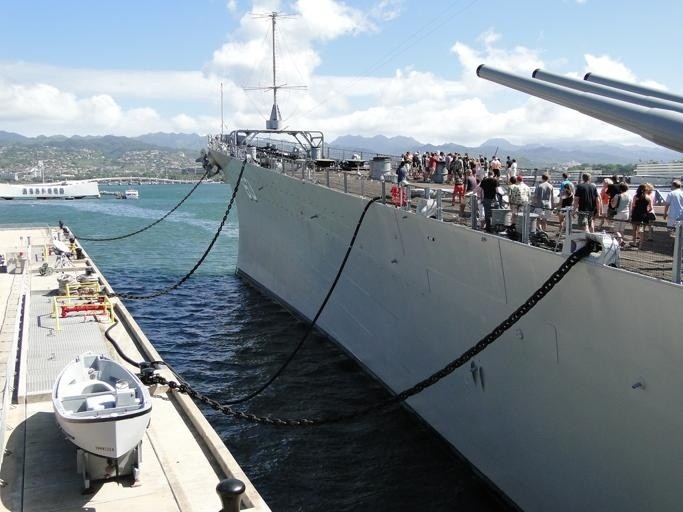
[209,11,683,512]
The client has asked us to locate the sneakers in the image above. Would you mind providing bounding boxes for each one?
[636,234,654,241]
[555,232,562,236]
[630,243,644,250]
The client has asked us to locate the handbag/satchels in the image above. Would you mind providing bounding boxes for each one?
[647,212,656,221]
[610,210,617,215]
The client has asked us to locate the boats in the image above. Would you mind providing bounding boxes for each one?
[51,351,152,460]
[126,190,140,200]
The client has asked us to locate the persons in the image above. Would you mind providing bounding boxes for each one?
[396,148,682,250]
